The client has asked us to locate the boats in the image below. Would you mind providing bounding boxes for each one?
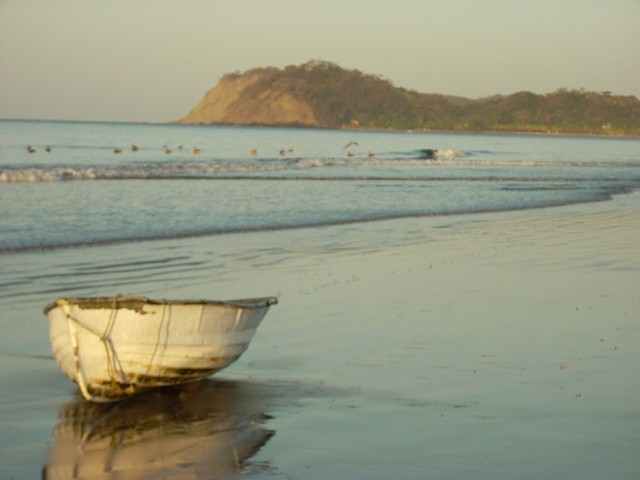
[44,295,278,403]
